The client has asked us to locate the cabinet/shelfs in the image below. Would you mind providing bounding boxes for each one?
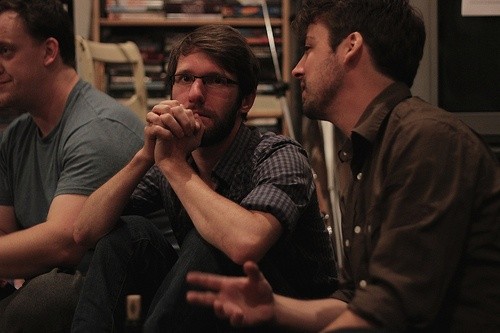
[90,0,303,146]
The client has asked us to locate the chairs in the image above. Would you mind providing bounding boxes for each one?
[77,34,148,125]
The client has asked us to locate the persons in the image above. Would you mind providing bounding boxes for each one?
[186,0,500,333]
[69,24,338,333]
[0,0,146,333]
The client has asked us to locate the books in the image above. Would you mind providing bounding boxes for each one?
[165,13,224,20]
[221,0,283,131]
[104,0,166,90]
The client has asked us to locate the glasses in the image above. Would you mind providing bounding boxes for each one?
[169,74,239,89]
